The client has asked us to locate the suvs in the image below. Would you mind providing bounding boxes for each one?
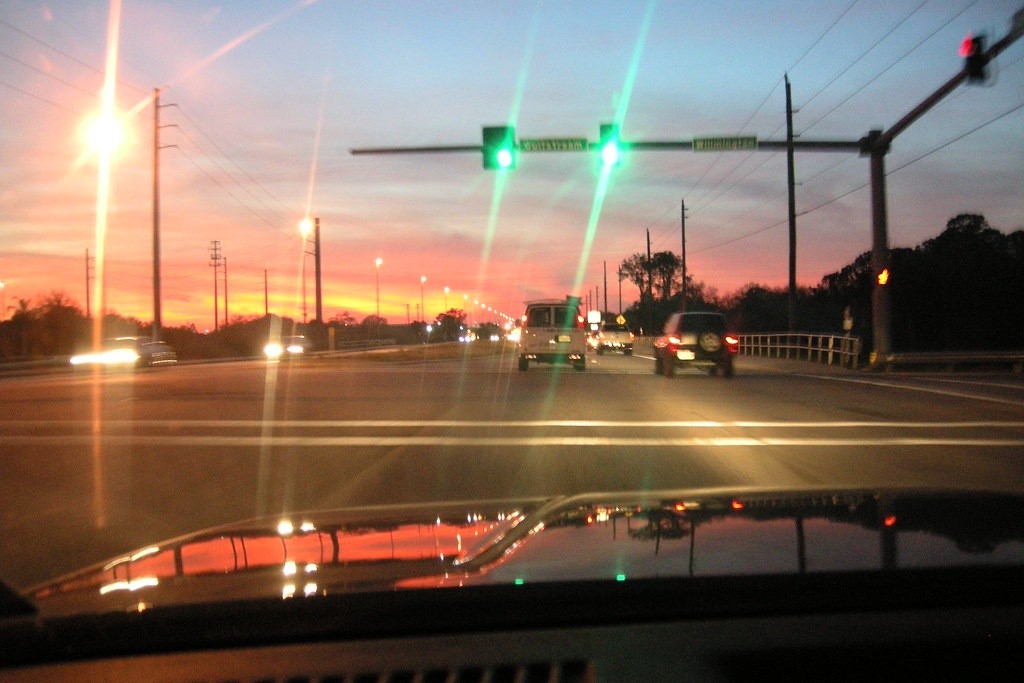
[653,312,739,379]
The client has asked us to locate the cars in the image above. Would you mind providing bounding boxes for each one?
[275,335,312,359]
[587,321,635,356]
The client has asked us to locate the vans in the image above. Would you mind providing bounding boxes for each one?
[518,297,589,372]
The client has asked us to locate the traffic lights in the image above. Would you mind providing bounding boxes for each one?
[962,33,991,85]
[600,124,624,171]
[482,126,517,172]
